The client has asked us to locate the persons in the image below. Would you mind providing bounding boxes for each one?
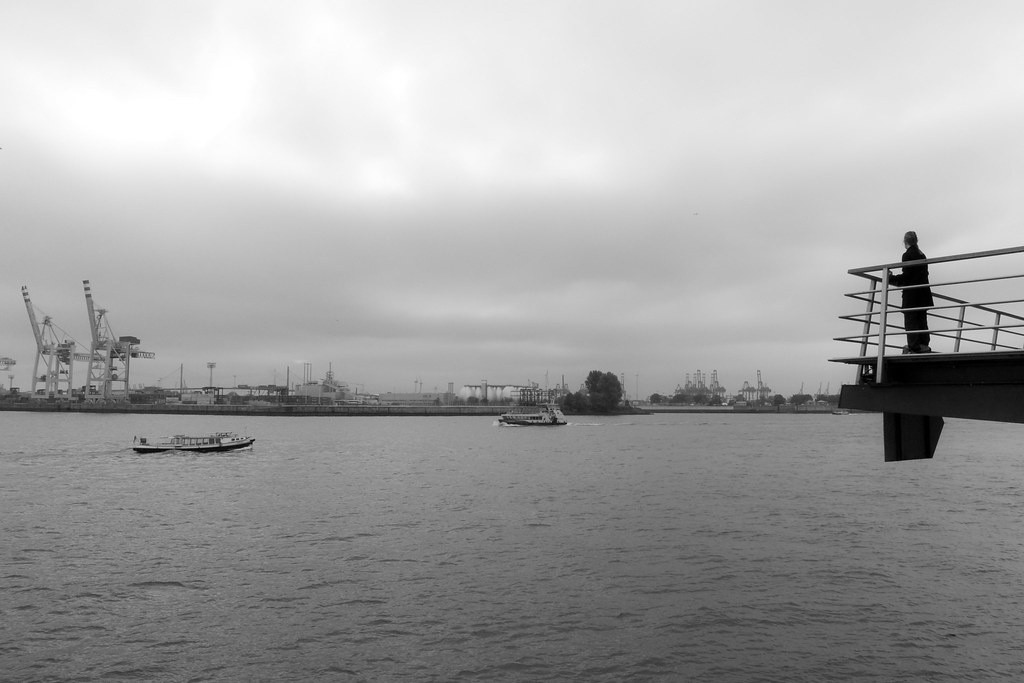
[888,231,934,354]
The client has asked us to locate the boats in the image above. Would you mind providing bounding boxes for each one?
[132,431,256,452]
[497,405,568,425]
